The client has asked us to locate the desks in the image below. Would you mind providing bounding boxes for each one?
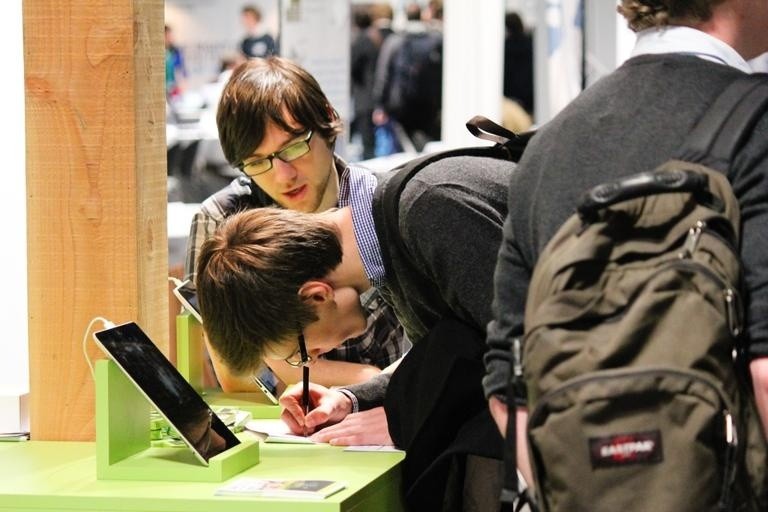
[0,390,405,512]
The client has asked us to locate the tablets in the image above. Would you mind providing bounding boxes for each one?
[92,321,241,468]
[172,277,287,404]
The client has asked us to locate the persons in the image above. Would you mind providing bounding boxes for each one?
[196,142,518,512]
[221,2,279,63]
[162,20,193,93]
[183,54,412,400]
[347,1,537,158]
[480,3,766,512]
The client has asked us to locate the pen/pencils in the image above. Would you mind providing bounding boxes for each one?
[303,366,309,437]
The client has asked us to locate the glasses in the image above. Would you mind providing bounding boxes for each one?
[238,127,315,177]
[285,315,317,369]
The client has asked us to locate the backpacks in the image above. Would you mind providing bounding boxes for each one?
[370,113,536,303]
[502,73,768,508]
